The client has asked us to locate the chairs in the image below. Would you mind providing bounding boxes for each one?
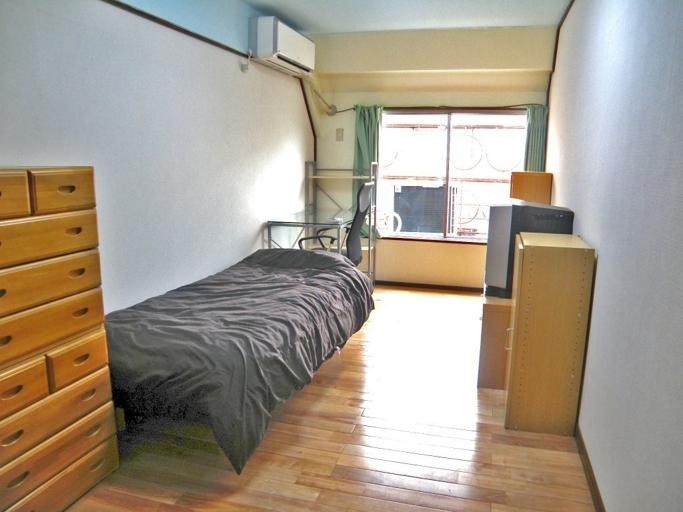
[298,182,375,266]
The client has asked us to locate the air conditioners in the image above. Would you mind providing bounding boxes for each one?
[241,16,318,79]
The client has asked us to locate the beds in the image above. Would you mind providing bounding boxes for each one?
[104,249,374,477]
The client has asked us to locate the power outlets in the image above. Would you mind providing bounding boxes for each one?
[336,129,342,141]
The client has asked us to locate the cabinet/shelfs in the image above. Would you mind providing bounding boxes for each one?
[477,294,512,390]
[0,167,120,511]
[305,161,379,286]
[510,172,552,206]
[504,232,595,438]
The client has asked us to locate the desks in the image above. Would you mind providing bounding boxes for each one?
[267,208,370,254]
